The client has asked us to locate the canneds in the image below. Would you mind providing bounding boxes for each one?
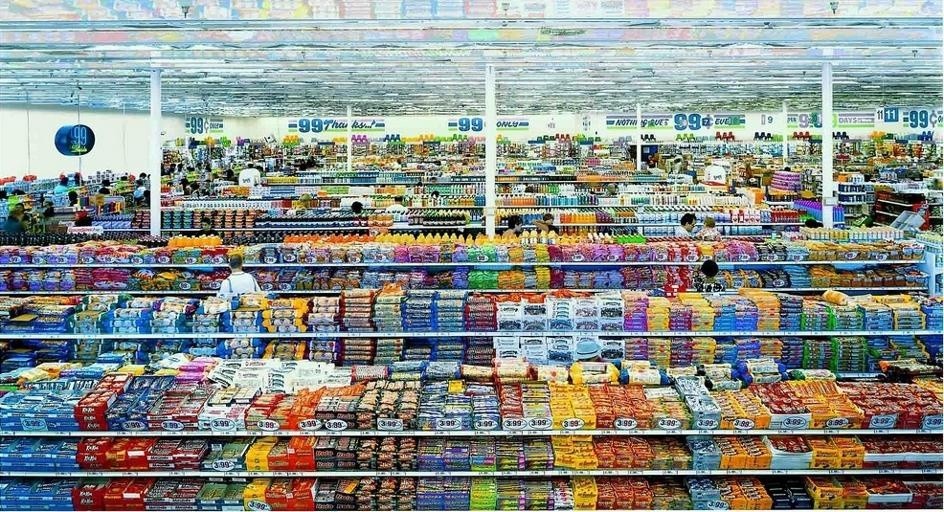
[800,226,848,240]
[849,226,903,241]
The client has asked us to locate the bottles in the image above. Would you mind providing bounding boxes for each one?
[284,230,376,243]
[222,231,282,245]
[255,214,368,228]
[547,207,635,223]
[366,210,407,226]
[169,233,222,247]
[482,208,546,226]
[616,233,645,244]
[181,197,271,209]
[407,208,481,226]
[0,231,103,245]
[474,193,536,207]
[102,231,168,248]
[437,196,474,208]
[376,230,465,243]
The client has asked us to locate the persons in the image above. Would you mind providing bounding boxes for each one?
[133,179,147,206]
[785,167,791,172]
[0,190,6,198]
[203,180,211,196]
[162,164,166,176]
[43,200,55,217]
[536,214,558,234]
[99,188,111,194]
[13,188,25,195]
[196,163,202,171]
[121,175,127,181]
[668,156,683,176]
[804,218,824,228]
[864,169,880,183]
[139,172,146,180]
[646,153,656,168]
[101,179,110,188]
[14,202,30,222]
[205,165,211,171]
[352,201,363,214]
[53,177,69,195]
[220,254,262,293]
[429,177,438,182]
[7,215,25,233]
[431,192,441,197]
[169,163,176,171]
[140,190,150,208]
[386,195,408,212]
[674,213,697,238]
[75,210,92,227]
[187,166,195,172]
[417,181,423,186]
[525,187,534,193]
[247,155,326,178]
[68,191,78,206]
[503,215,523,235]
[175,164,183,174]
[696,217,722,242]
[168,168,175,174]
[226,169,238,182]
[190,182,200,197]
[196,216,220,236]
[697,259,725,292]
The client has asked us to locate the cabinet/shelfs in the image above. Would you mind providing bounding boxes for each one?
[875,185,927,232]
[1,246,942,355]
[836,182,868,226]
[136,175,807,232]
[0,363,942,504]
[929,201,944,235]
